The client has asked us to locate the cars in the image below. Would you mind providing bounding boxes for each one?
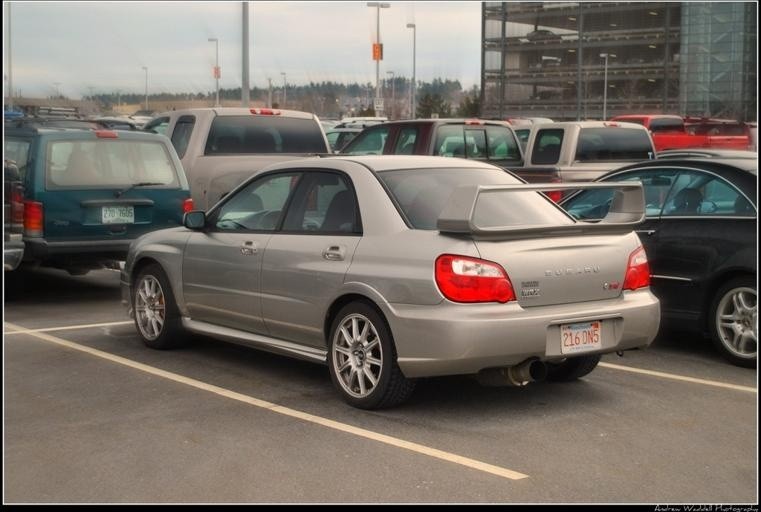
[527,30,561,41]
[4,106,757,411]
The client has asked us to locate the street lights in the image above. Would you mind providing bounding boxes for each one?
[600,53,616,120]
[406,23,416,106]
[141,65,149,106]
[207,38,219,105]
[366,1,390,114]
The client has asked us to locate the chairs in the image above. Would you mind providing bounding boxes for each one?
[403,186,444,228]
[444,141,476,156]
[674,189,707,216]
[216,127,278,153]
[317,190,355,232]
